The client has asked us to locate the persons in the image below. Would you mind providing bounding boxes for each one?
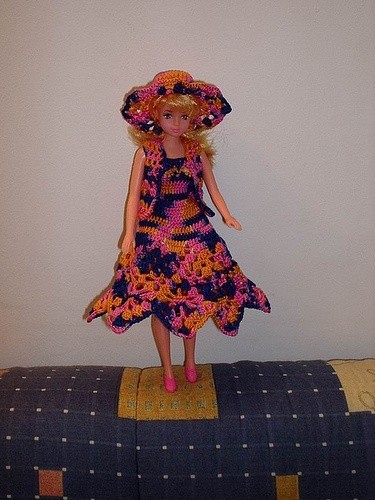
[85,69,272,394]
